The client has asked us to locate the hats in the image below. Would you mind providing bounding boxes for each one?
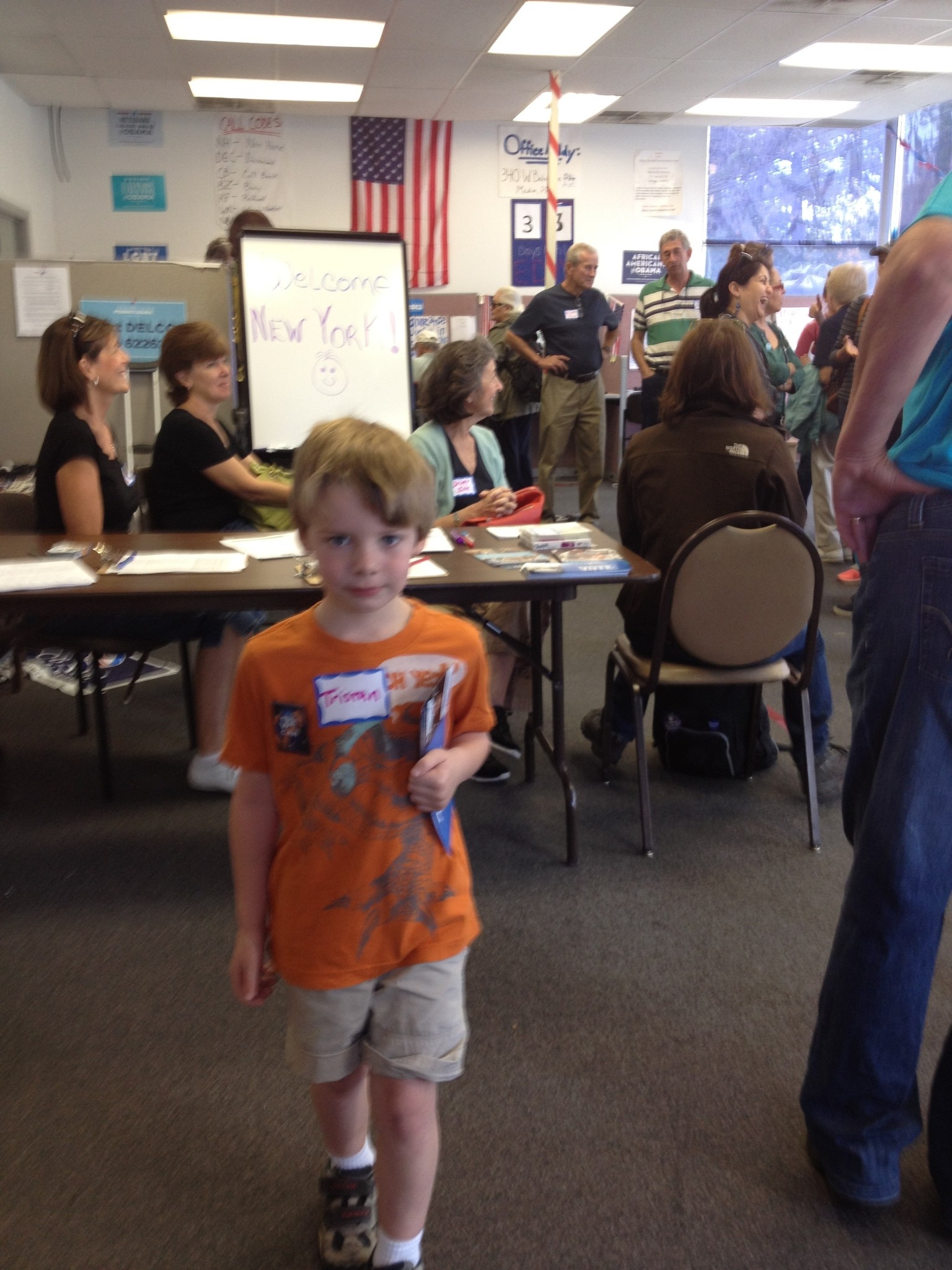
[869,237,898,256]
[410,331,440,349]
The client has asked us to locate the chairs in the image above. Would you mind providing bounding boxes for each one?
[606,508,823,858]
[0,490,198,796]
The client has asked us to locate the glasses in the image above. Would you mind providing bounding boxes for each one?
[730,251,752,281]
[761,244,771,254]
[68,307,86,337]
[492,302,501,309]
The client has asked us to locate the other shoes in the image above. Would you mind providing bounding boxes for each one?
[807,1134,893,1225]
[799,760,841,804]
[837,568,861,581]
[817,543,853,563]
[487,706,521,759]
[469,755,511,782]
[832,592,860,617]
[580,707,627,773]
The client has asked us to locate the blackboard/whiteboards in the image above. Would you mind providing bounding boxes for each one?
[239,224,418,456]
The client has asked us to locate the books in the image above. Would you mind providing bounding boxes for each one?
[519,521,593,550]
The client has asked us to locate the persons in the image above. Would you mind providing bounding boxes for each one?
[406,339,526,782]
[208,210,271,263]
[35,313,142,540]
[144,322,294,795]
[482,229,906,805]
[410,330,445,423]
[219,419,495,1270]
[800,154,952,1225]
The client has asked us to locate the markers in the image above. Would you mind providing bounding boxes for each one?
[450,530,476,548]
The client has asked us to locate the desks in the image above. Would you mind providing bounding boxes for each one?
[1,520,661,868]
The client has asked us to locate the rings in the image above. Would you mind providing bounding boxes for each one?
[852,517,861,520]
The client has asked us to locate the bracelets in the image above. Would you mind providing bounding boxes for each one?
[453,511,460,527]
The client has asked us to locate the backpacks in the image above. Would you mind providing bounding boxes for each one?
[503,322,542,402]
[652,675,777,781]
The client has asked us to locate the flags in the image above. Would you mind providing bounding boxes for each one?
[348,117,452,287]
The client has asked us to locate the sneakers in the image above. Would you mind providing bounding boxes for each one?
[187,752,239,794]
[319,1155,376,1270]
